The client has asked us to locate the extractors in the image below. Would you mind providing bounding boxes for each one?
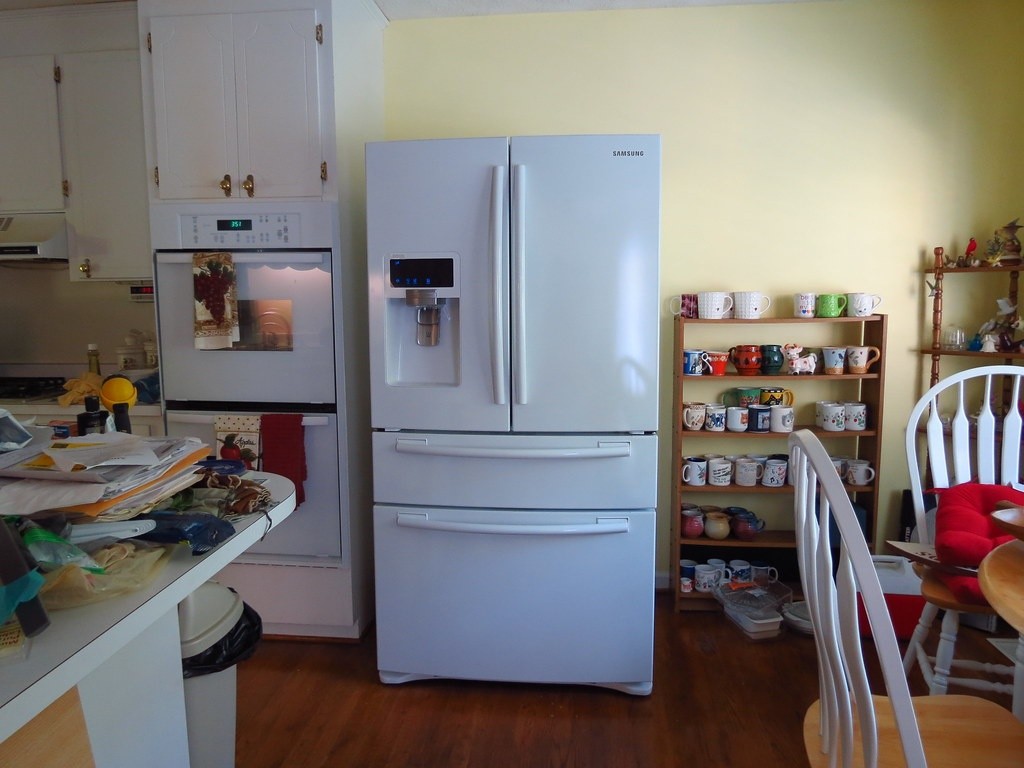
[0,211,69,262]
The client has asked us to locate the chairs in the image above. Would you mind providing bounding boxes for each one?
[788,429,1024,768]
[906,366,1024,695]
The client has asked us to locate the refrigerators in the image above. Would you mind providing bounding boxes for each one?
[362,132,662,698]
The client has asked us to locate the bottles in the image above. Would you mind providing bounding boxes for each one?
[77,395,109,435]
[87,343,101,376]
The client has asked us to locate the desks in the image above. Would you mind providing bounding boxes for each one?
[0,471,295,768]
[978,539,1024,722]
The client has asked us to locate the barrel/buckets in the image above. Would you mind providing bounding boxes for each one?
[115,341,158,384]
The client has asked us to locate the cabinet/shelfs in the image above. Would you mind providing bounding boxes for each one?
[0,0,391,281]
[920,246,1024,489]
[672,314,887,620]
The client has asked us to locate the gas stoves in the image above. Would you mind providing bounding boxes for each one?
[0,376,68,403]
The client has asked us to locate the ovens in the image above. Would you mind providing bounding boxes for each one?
[145,199,368,625]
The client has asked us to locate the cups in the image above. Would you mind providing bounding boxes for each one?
[792,290,882,318]
[814,399,867,432]
[681,452,789,486]
[788,452,875,487]
[679,558,778,593]
[682,385,795,433]
[681,502,766,540]
[668,291,771,320]
[684,343,880,378]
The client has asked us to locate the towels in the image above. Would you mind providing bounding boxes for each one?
[214,414,261,470]
[260,412,307,511]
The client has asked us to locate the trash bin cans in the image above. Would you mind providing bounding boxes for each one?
[178,580,262,768]
